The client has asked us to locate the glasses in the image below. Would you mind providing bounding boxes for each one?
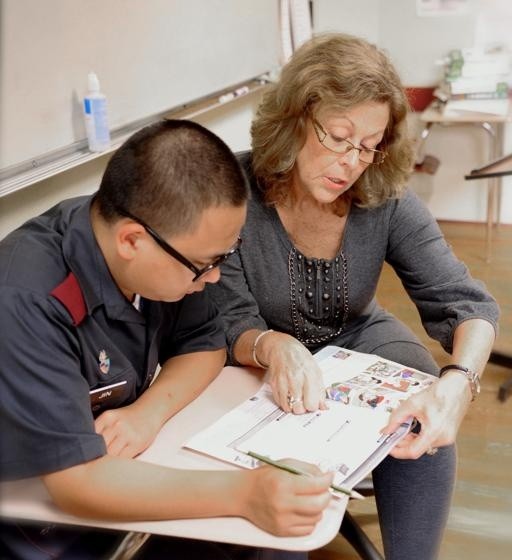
[304,108,389,165]
[115,205,242,283]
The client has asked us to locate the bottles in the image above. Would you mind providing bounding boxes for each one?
[82,72,111,154]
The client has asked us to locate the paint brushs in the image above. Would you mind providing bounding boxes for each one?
[234,446,366,499]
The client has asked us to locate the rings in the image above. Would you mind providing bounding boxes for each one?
[288,393,303,409]
[427,441,438,457]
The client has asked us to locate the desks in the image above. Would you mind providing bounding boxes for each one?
[414,93,509,262]
[0,362,355,559]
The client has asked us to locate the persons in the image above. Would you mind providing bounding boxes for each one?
[208,31,503,560]
[1,116,335,558]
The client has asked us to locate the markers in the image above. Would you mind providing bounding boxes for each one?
[219,87,248,103]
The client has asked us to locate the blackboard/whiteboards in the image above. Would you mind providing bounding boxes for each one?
[0,2,294,197]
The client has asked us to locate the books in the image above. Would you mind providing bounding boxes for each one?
[183,343,441,500]
[433,51,510,121]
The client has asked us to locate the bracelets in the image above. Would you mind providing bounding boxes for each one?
[252,329,274,370]
[438,363,481,402]
